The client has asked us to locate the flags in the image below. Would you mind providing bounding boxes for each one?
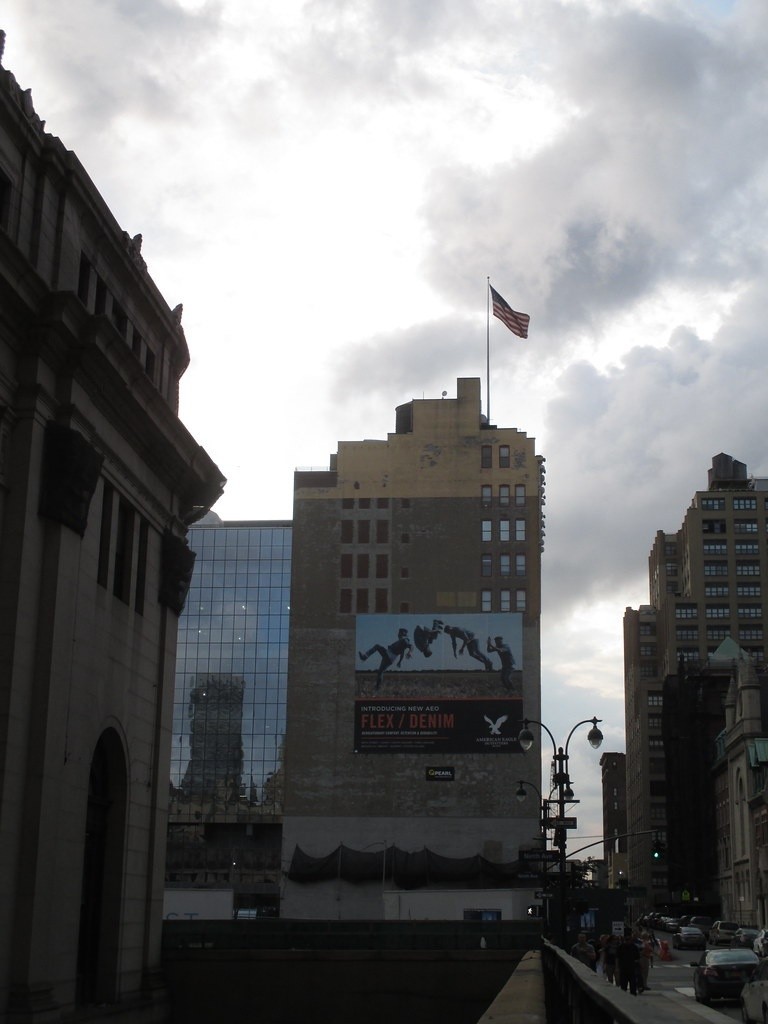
[490,285,529,338]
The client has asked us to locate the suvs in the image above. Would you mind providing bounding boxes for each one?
[708,920,739,946]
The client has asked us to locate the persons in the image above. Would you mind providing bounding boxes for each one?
[572,920,657,995]
[414,620,443,656]
[444,625,493,670]
[487,636,516,690]
[360,629,414,689]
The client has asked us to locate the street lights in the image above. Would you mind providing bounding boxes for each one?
[517,717,605,937]
[512,779,575,943]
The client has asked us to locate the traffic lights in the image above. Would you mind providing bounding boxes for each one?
[527,904,546,917]
[649,840,667,869]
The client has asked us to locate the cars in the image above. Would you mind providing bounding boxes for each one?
[740,957,768,1024]
[753,928,768,957]
[672,927,706,951]
[690,948,761,1004]
[637,911,712,936]
[730,927,759,948]
